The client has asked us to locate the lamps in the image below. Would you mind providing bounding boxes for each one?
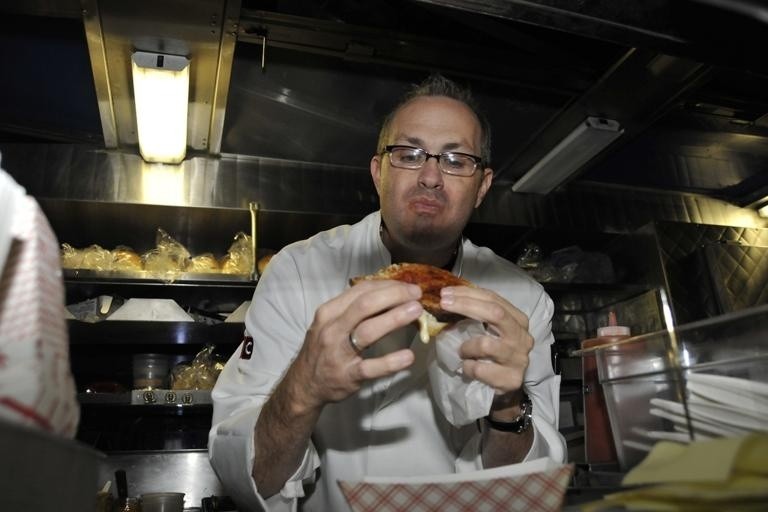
[130,52,191,165]
[511,117,625,196]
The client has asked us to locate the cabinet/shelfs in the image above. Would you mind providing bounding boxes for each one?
[36,198,678,407]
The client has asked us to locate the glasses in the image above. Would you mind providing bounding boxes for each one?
[381,144,484,177]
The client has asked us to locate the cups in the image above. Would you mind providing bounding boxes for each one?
[94,492,113,512]
[593,331,713,472]
[115,497,140,512]
[138,490,185,512]
[200,495,233,512]
[130,352,171,392]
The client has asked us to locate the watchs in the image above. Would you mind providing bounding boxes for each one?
[488,389,532,434]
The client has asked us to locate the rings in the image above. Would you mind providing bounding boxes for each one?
[349,331,369,353]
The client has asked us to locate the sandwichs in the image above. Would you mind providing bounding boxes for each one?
[351,259,481,344]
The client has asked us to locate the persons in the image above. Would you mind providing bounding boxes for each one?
[206,74,569,512]
[0,151,81,439]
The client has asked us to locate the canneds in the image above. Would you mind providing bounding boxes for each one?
[135,354,172,390]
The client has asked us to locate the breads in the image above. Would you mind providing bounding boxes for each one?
[64,248,273,275]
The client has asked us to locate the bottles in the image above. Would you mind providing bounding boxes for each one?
[580,308,647,468]
